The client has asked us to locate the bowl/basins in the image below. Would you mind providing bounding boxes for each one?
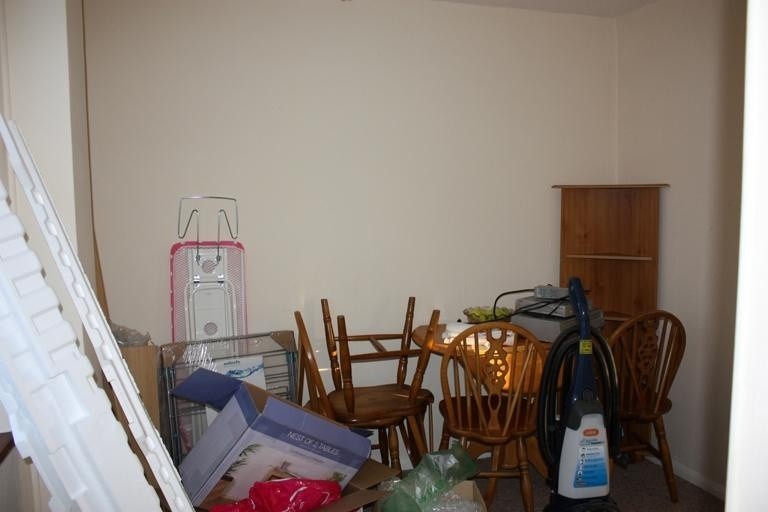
[461,305,512,325]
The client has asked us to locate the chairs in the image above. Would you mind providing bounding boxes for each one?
[442,321,548,510]
[590,308,687,503]
[292,295,441,483]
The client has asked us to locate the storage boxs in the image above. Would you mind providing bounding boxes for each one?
[100,342,490,510]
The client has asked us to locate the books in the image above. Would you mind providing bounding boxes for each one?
[442,320,514,339]
[443,333,516,347]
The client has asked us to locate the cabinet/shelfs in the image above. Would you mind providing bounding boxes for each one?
[548,180,672,458]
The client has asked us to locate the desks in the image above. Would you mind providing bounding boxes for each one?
[412,320,576,490]
